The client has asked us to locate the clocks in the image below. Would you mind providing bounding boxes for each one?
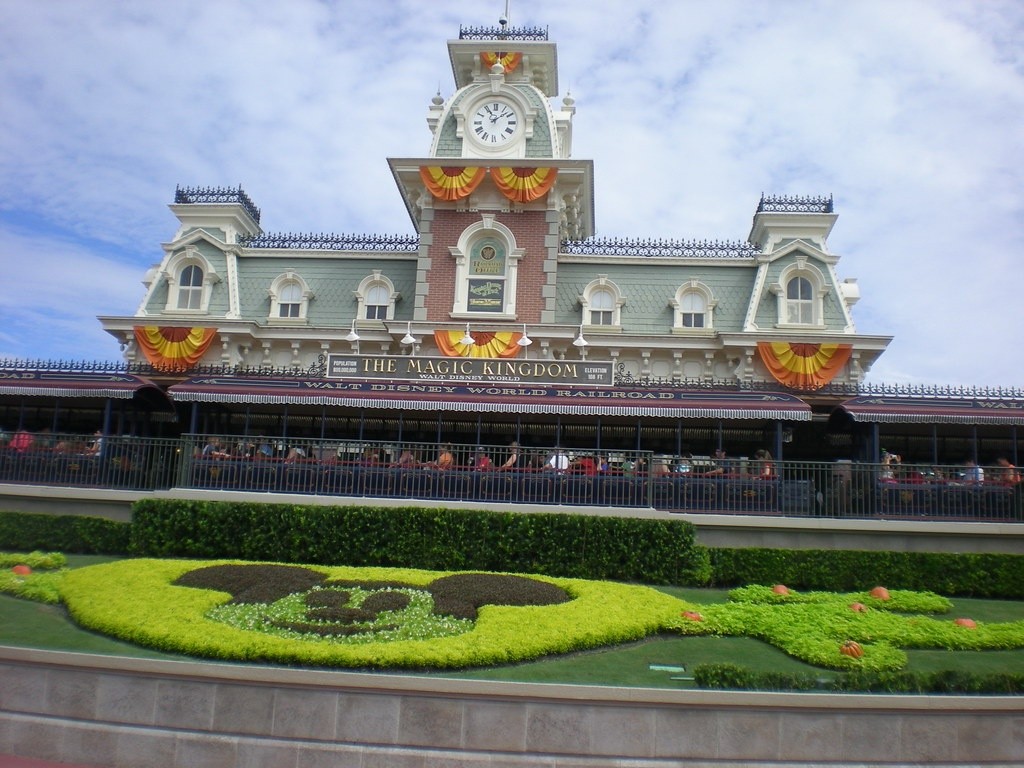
[465,96,525,152]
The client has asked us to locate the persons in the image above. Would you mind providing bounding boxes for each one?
[472,447,494,472]
[497,442,529,473]
[960,455,984,485]
[427,441,453,470]
[702,446,732,479]
[880,459,900,484]
[754,450,778,480]
[573,452,694,477]
[526,451,551,472]
[195,434,421,469]
[540,444,571,475]
[0,428,102,459]
[996,454,1022,488]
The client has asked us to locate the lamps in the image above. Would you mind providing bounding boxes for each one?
[400,321,415,356]
[345,319,360,354]
[516,323,533,359]
[459,323,475,357]
[572,325,587,360]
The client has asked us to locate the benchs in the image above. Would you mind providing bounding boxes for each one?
[0,451,108,486]
[191,459,781,513]
[876,483,1024,518]
